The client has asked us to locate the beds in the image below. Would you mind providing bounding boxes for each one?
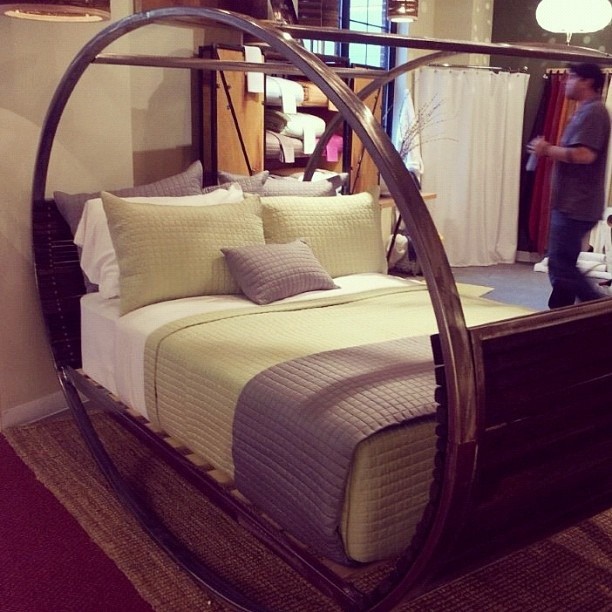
[27,6,612,610]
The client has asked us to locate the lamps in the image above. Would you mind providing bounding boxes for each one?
[386,0,418,24]
[0,0,111,23]
[535,0,612,46]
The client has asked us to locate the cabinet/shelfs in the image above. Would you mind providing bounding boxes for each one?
[199,42,386,201]
[132,0,339,46]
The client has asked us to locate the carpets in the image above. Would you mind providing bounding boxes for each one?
[0,398,612,612]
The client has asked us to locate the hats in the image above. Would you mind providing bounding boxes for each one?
[566,61,604,88]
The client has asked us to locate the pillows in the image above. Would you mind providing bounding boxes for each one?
[219,238,342,306]
[260,173,341,196]
[202,169,270,198]
[54,160,204,295]
[74,186,243,300]
[99,188,265,315]
[259,192,387,278]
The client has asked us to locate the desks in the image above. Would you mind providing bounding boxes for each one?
[380,191,437,260]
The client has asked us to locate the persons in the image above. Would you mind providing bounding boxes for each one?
[524,62,612,309]
